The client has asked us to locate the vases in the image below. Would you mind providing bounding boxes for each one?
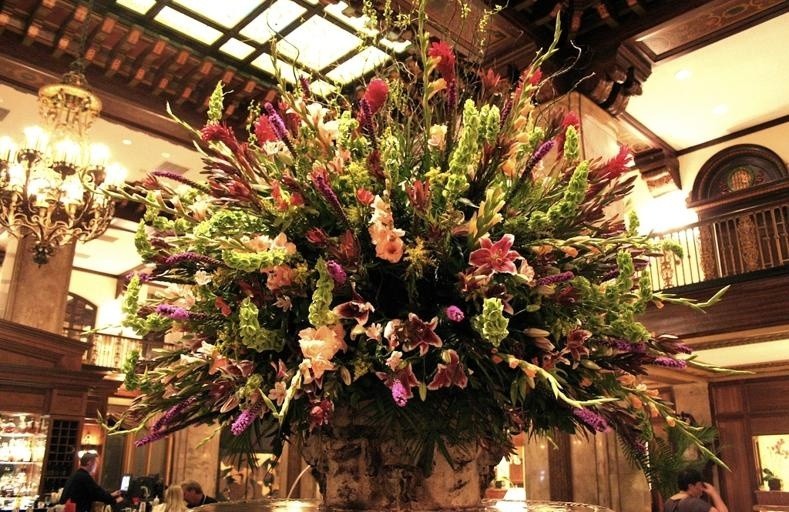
[313,426,481,512]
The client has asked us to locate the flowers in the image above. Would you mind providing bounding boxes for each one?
[78,0,757,501]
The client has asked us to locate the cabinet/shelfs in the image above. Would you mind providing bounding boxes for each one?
[0,411,54,512]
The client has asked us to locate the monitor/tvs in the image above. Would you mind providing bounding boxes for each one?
[120,472,133,495]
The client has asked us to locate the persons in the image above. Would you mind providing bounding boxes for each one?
[47,453,217,512]
[663,469,729,512]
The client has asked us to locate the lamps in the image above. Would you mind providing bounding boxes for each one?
[0,0,128,268]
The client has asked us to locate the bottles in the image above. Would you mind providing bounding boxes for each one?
[152,495,160,510]
[4,417,16,432]
